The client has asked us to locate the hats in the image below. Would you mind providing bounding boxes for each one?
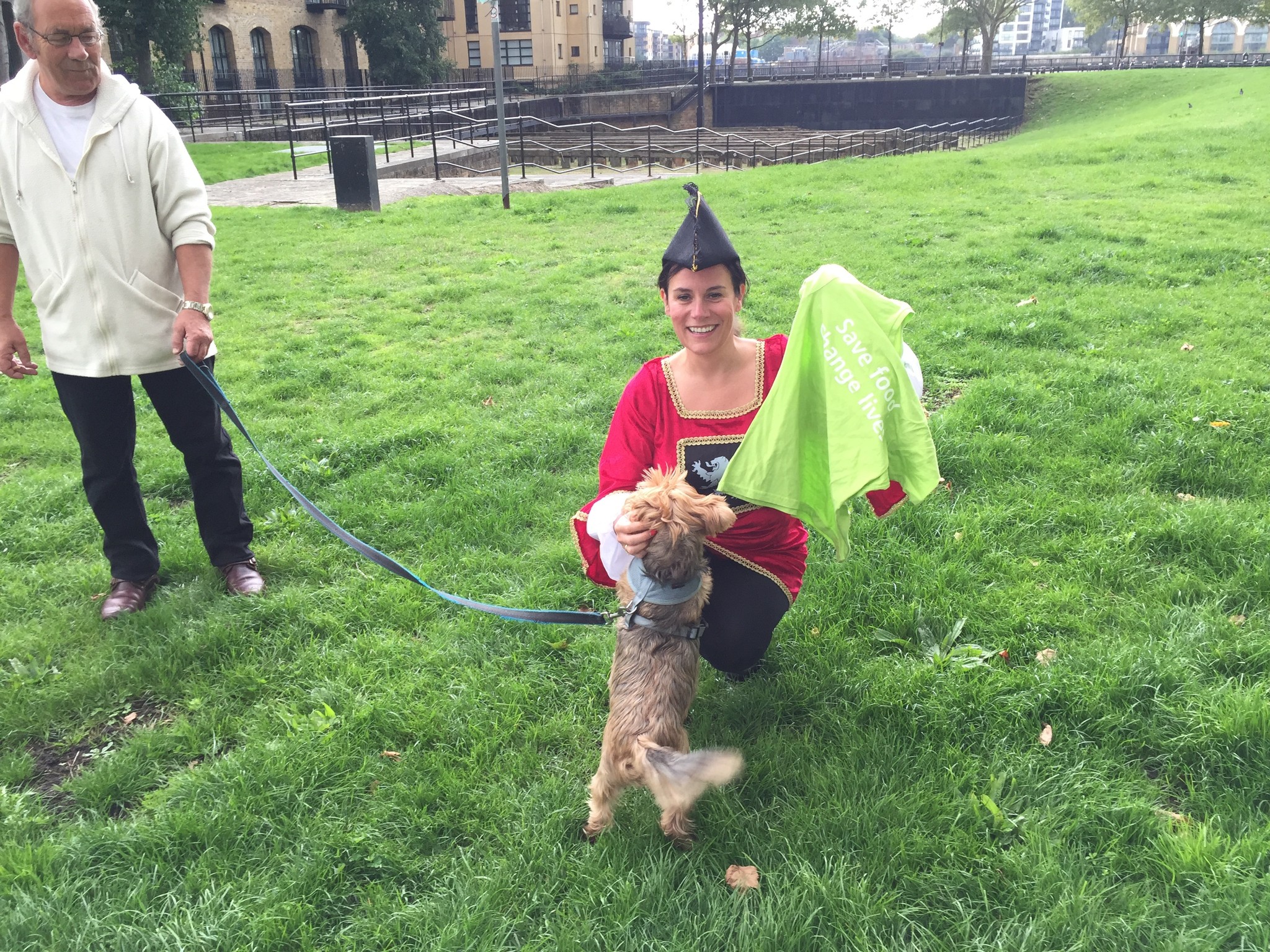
[663,179,739,272]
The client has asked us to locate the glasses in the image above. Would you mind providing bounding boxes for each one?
[27,25,106,47]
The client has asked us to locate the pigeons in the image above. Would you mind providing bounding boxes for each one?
[1239,88,1244,95]
[1188,103,1192,108]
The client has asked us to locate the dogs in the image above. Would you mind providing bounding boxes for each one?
[578,456,749,851]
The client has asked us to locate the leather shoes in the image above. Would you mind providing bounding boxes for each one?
[219,555,267,600]
[101,575,159,622]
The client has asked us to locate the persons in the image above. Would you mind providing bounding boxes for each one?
[568,183,925,683]
[0,0,265,621]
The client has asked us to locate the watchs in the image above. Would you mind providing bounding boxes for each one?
[182,301,214,321]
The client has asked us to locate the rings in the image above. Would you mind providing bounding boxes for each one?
[625,545,628,553]
